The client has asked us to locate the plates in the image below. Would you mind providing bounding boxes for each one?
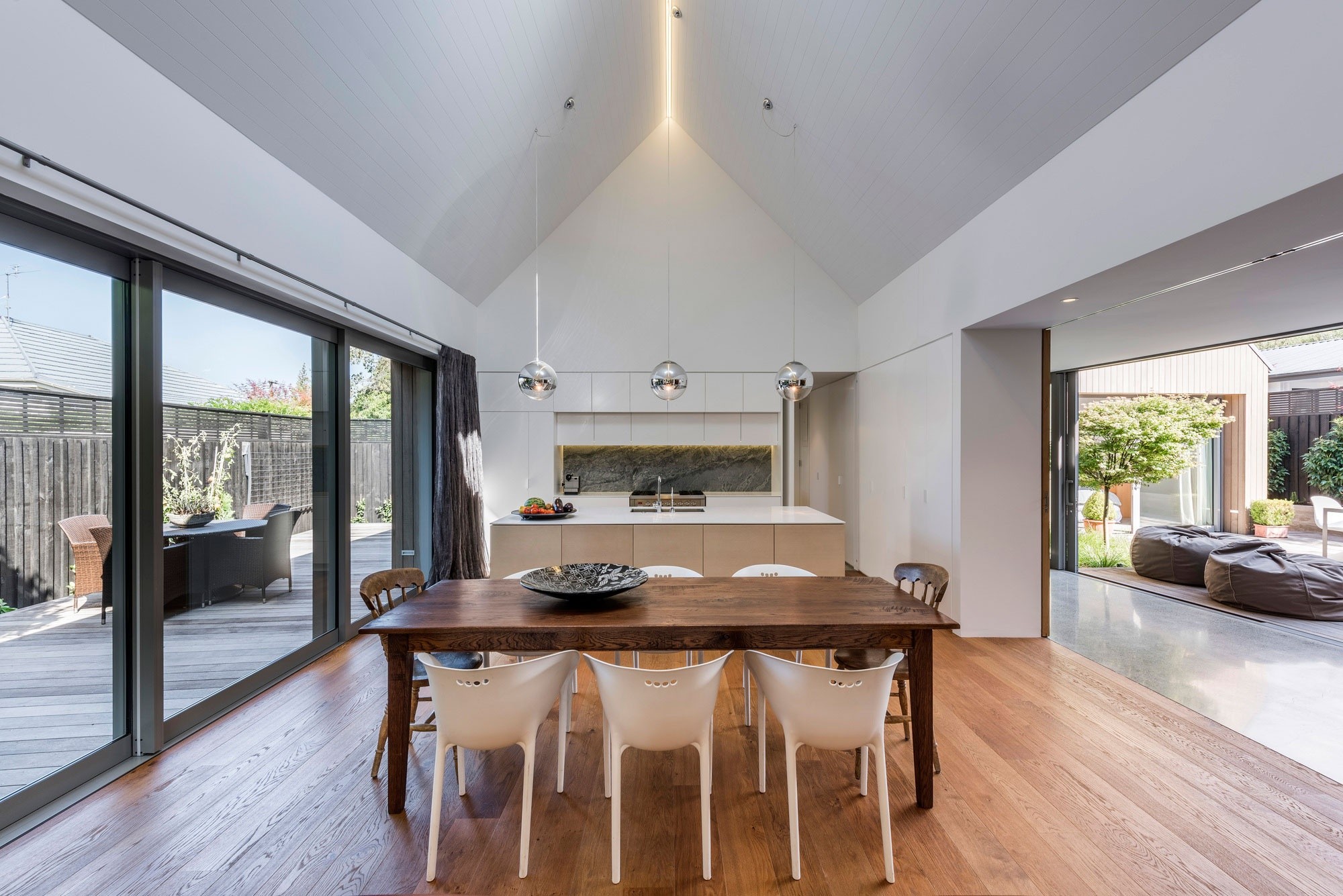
[520,562,649,603]
[511,508,577,520]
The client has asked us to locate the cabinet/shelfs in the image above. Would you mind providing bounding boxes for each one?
[477,372,786,579]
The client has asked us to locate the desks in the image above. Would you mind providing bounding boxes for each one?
[159,518,269,608]
[359,576,960,816]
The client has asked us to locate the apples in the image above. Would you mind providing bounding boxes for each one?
[524,504,556,514]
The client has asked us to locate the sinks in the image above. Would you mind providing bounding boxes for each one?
[670,506,706,513]
[629,506,662,513]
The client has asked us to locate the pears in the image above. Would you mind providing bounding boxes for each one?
[519,506,525,513]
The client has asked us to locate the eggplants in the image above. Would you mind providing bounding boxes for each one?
[553,498,573,513]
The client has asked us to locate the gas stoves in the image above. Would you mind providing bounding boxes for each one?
[628,490,706,507]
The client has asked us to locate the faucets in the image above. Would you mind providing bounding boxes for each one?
[652,476,663,513]
[671,487,673,512]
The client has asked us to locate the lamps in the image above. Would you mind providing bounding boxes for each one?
[517,97,577,402]
[649,7,688,400]
[760,98,813,403]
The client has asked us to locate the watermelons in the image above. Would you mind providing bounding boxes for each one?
[524,497,545,509]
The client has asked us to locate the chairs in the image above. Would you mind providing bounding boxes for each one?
[62,502,302,625]
[1311,496,1343,558]
[612,565,706,670]
[833,562,949,782]
[729,563,818,725]
[359,568,483,784]
[418,568,579,882]
[743,650,905,884]
[582,649,736,885]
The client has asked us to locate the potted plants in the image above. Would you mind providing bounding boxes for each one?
[1249,496,1295,538]
[1082,489,1117,535]
[163,422,243,528]
